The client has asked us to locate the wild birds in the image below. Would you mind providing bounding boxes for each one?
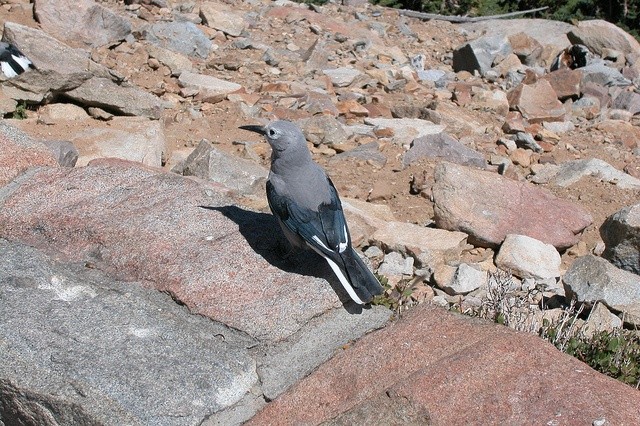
[237,119,388,307]
